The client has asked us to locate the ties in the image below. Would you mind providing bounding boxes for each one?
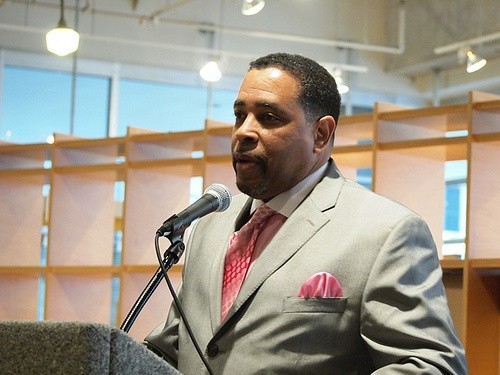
[221,204,275,325]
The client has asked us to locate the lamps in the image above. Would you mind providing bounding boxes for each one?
[46,0,80,58]
[333,69,350,94]
[465,49,487,73]
[199,32,222,83]
[240,0,265,16]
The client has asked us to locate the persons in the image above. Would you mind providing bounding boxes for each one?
[141,52,466,375]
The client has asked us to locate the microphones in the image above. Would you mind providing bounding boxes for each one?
[156,183,232,236]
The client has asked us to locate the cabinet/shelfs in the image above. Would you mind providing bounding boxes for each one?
[0,91,500,375]
